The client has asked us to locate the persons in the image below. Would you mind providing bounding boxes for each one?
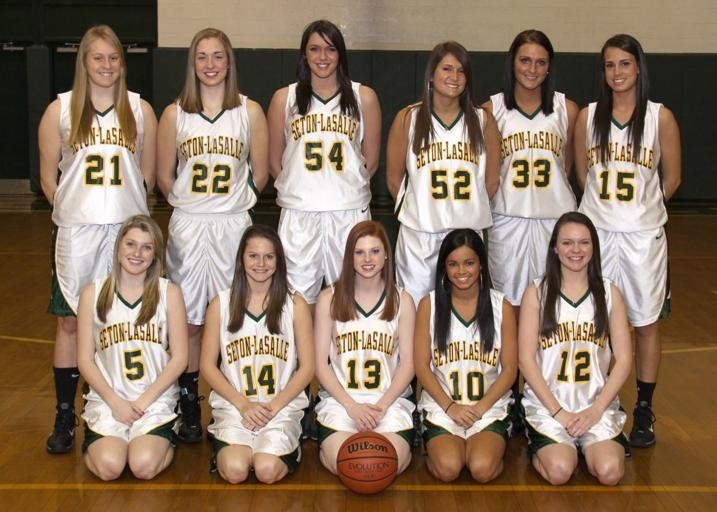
[312,221,418,478]
[386,38,502,311]
[413,228,518,484]
[517,211,634,486]
[37,24,158,454]
[76,214,189,482]
[481,28,580,310]
[158,28,270,447]
[196,226,314,485]
[574,33,684,449]
[265,18,384,439]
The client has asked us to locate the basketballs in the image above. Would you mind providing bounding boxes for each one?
[337,431,398,494]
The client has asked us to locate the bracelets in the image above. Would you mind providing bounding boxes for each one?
[445,402,457,414]
[553,408,563,419]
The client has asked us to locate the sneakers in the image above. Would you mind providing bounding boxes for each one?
[310,391,319,440]
[47,405,80,453]
[300,393,312,439]
[631,407,656,448]
[179,395,205,445]
[411,411,420,447]
